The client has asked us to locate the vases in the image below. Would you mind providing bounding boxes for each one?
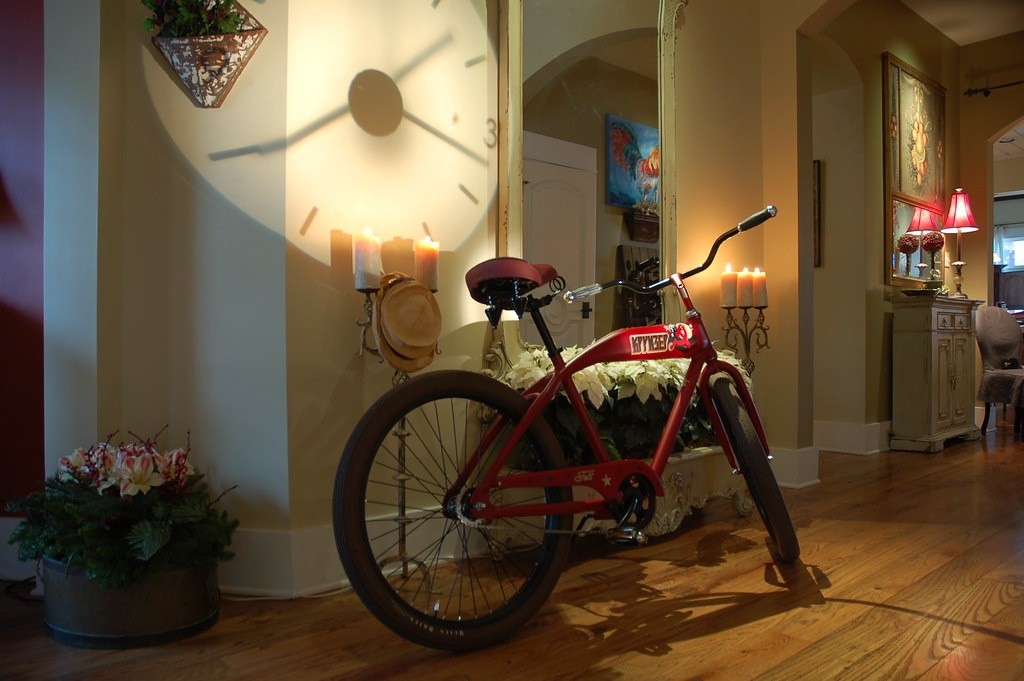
[488,446,756,561]
[623,213,661,242]
[930,251,936,278]
[35,530,227,651]
[903,251,913,274]
[153,26,265,107]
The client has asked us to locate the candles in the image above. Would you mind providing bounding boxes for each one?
[720,261,738,308]
[352,226,382,288]
[750,265,767,307]
[736,265,752,307]
[413,232,444,286]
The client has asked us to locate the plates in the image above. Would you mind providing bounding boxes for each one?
[900,289,938,295]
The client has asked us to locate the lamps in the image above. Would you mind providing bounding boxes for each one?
[941,187,980,297]
[904,207,936,277]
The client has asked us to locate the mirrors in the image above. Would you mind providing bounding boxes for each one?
[891,197,946,280]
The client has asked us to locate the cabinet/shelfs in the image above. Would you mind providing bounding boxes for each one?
[886,297,987,453]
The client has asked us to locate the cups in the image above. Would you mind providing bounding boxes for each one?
[997,302,1006,308]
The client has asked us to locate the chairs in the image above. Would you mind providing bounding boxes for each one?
[974,306,1024,440]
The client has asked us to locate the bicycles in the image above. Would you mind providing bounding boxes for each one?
[331,204,802,654]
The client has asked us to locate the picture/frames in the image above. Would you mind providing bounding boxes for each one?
[814,158,821,268]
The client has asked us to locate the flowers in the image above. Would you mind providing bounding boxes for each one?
[498,340,751,459]
[9,416,242,574]
[897,234,918,253]
[921,232,945,253]
[139,1,254,42]
[632,185,661,216]
